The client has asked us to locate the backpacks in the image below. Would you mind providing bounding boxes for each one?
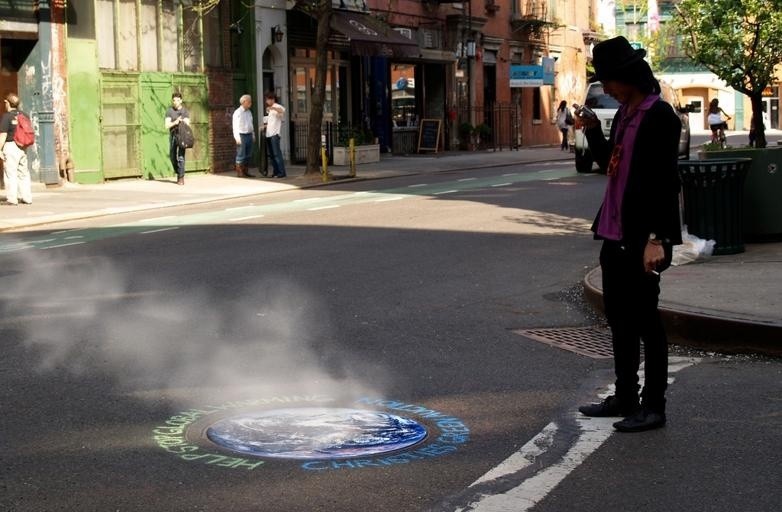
[12,112,36,150]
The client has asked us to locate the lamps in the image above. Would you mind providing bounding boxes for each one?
[462,28,476,61]
[271,24,284,46]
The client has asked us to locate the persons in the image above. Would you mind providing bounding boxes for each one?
[573,36,685,434]
[0,91,33,205]
[708,99,731,143]
[164,92,191,187]
[232,94,257,178]
[261,92,287,179]
[556,100,571,151]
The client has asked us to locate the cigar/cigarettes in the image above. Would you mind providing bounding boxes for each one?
[650,270,659,275]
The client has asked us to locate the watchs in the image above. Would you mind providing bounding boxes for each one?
[648,232,662,241]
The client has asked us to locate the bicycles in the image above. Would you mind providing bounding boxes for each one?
[708,117,730,149]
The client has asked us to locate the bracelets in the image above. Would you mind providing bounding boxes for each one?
[647,237,662,245]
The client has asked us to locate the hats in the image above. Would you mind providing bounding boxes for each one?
[585,35,646,83]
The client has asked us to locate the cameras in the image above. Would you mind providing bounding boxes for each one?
[577,105,595,118]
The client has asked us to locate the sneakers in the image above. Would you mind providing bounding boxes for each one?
[0,197,31,207]
[175,174,185,186]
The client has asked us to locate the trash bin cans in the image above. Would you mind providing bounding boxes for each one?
[677,158,752,255]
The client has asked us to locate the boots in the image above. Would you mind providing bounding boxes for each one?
[578,372,641,418]
[234,161,256,179]
[611,376,669,434]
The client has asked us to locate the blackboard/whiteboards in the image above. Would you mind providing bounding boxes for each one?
[418,119,442,150]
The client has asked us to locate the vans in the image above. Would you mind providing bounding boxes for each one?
[573,80,692,173]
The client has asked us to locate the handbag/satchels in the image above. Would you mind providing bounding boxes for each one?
[564,107,576,126]
[176,119,195,149]
